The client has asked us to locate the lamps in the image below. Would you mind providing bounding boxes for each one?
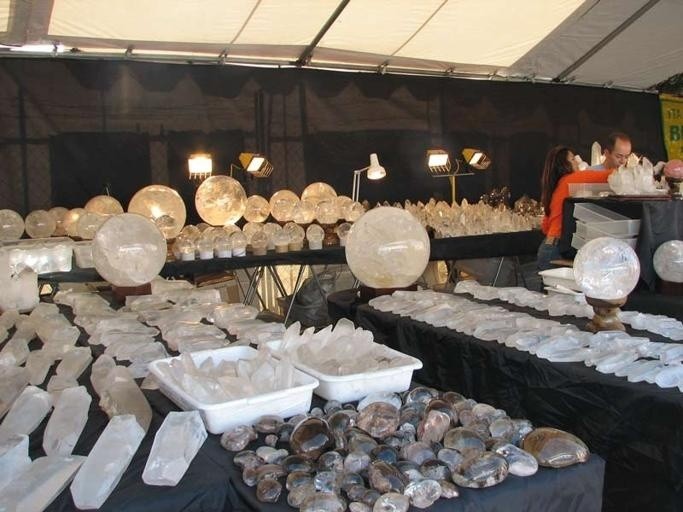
[426,147,491,204]
[352,154,386,203]
[229,153,274,181]
[187,153,213,187]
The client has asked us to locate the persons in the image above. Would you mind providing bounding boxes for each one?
[586,131,632,170]
[537,145,667,271]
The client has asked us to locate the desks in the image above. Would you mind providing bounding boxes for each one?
[0,233,683,512]
